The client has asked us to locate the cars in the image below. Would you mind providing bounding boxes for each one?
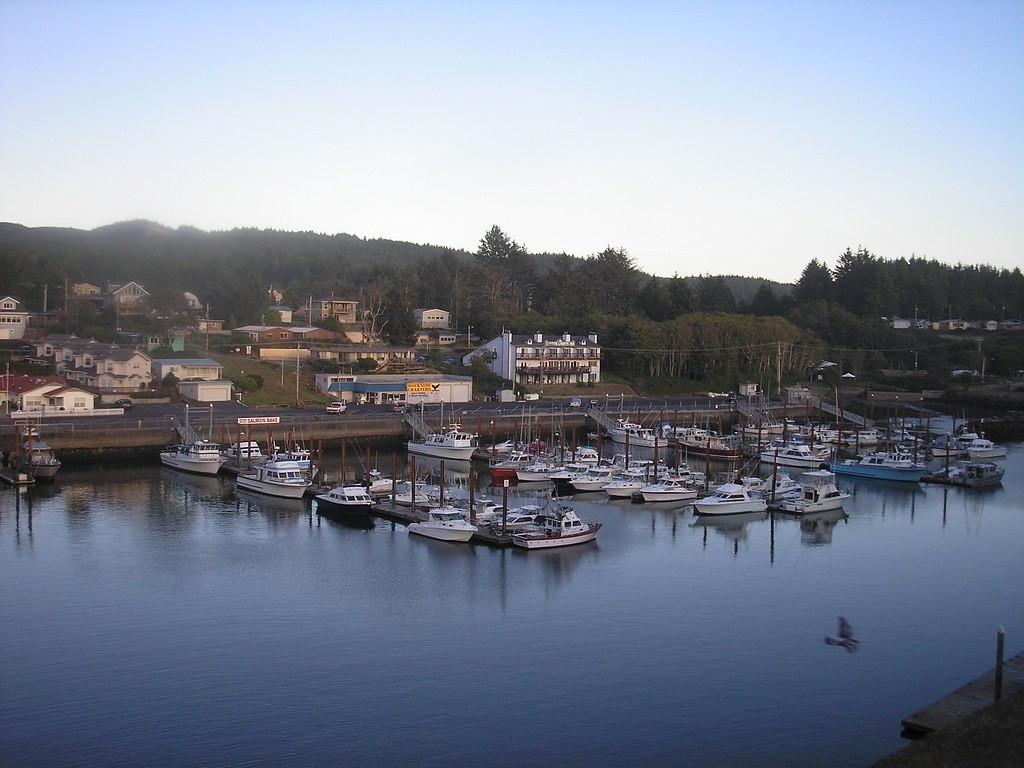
[326,401,347,416]
[570,396,581,407]
[115,399,133,410]
[393,402,411,414]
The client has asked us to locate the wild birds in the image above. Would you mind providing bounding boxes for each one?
[824,617,860,652]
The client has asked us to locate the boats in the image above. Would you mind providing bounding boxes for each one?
[222,381,1008,550]
[160,440,228,476]
[408,509,479,542]
[315,486,372,514]
[407,423,479,460]
[236,459,313,499]
[6,411,62,479]
